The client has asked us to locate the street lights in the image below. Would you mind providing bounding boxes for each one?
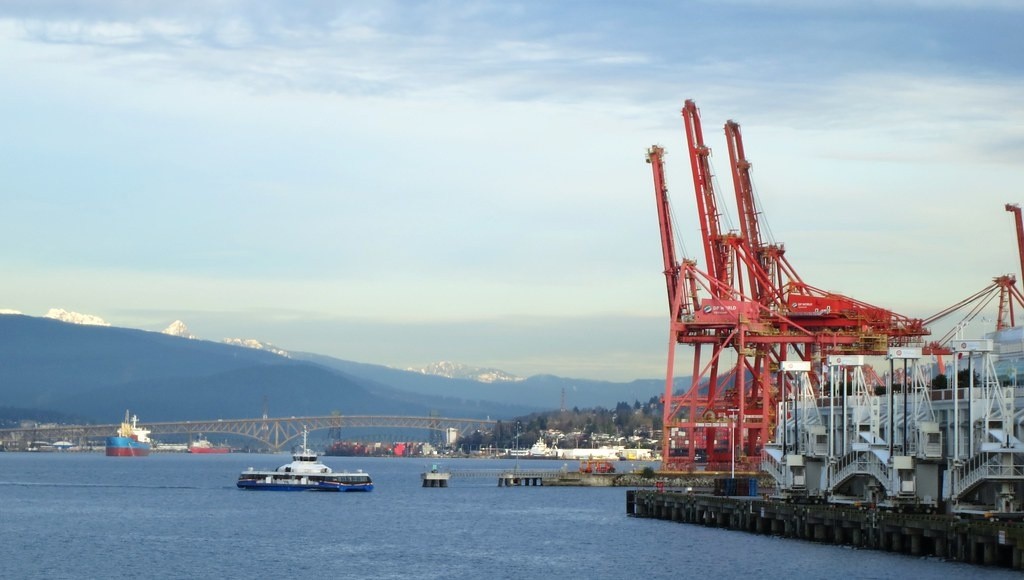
[512,420,522,469]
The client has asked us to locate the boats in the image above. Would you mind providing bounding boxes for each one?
[236,425,374,492]
[106,410,152,457]
[187,432,229,453]
